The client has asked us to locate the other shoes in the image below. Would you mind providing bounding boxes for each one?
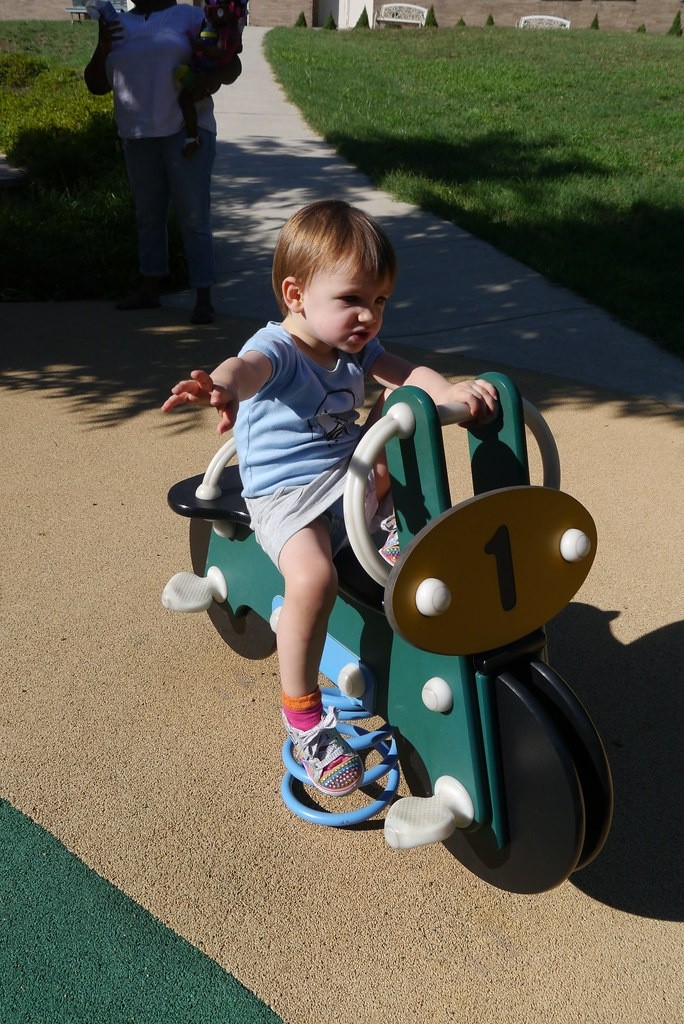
[182,138,200,158]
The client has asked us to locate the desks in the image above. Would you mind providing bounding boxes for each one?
[66,7,87,24]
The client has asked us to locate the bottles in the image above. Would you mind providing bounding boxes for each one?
[94,0,129,43]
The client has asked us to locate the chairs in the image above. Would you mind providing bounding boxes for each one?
[515,15,570,29]
[374,3,428,28]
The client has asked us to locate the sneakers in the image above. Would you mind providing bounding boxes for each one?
[378,515,400,567]
[281,707,364,797]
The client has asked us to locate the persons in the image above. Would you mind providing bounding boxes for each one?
[82,1,244,323]
[160,198,502,797]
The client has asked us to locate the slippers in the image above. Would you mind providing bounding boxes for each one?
[191,304,214,324]
[116,290,162,310]
